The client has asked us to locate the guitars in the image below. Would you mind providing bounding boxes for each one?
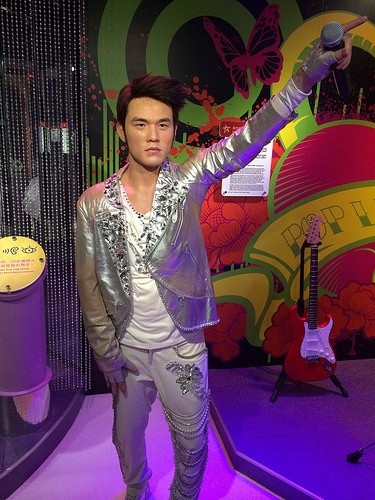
[286,212,337,382]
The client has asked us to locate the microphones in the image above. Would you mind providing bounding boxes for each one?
[320,22,352,97]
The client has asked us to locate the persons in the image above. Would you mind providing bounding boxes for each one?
[75,16,367,500]
